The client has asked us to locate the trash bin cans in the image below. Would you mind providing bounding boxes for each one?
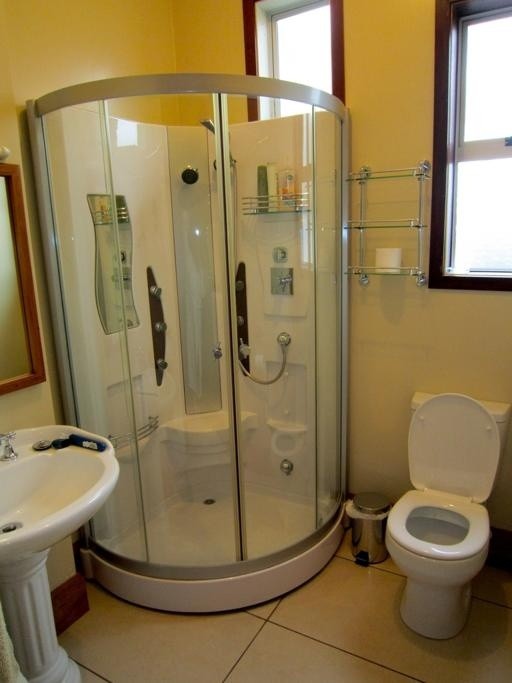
[347,492,393,564]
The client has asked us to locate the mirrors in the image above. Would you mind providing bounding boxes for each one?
[0,160,48,397]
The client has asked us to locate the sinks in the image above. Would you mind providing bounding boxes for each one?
[0,425,119,562]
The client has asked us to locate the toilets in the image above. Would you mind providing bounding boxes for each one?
[389,392,509,639]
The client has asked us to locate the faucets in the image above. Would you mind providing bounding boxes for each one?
[2,428,15,457]
